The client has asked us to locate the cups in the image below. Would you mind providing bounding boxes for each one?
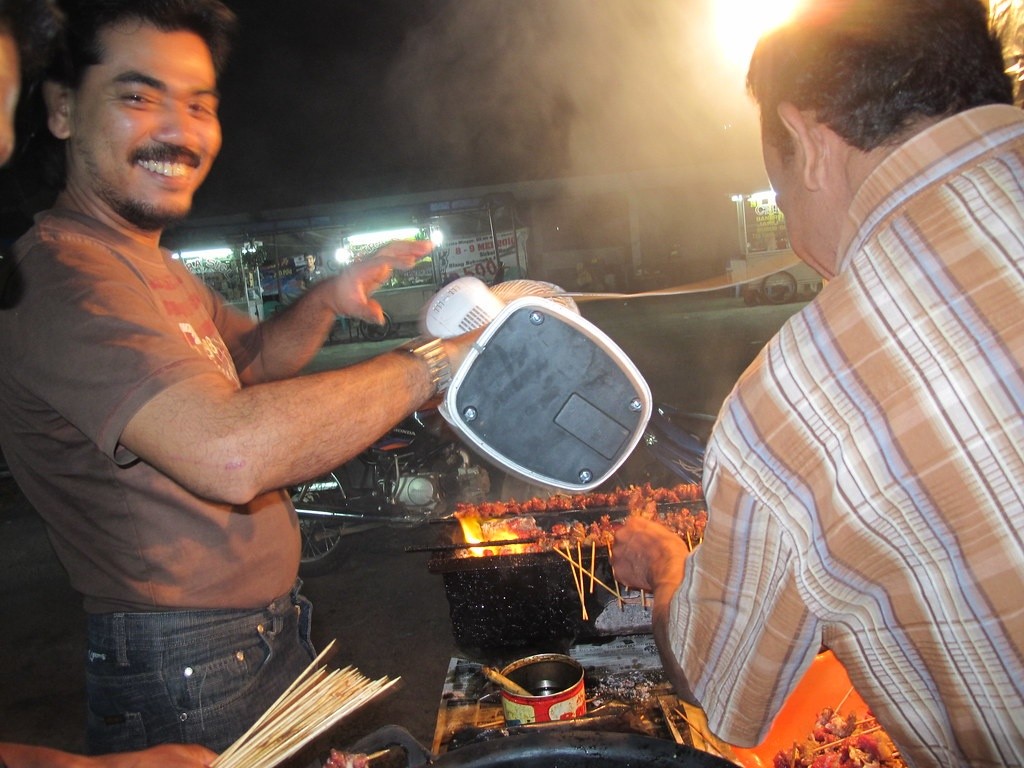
[499,653,587,727]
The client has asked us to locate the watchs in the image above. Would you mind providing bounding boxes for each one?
[397,336,452,397]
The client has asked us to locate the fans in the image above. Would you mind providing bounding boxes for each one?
[417,276,652,492]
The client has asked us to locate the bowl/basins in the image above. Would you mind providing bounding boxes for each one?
[731,650,869,768]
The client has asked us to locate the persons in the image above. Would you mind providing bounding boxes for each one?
[297,249,327,287]
[0,0,219,768]
[0,0,490,766]
[607,0,1024,765]
